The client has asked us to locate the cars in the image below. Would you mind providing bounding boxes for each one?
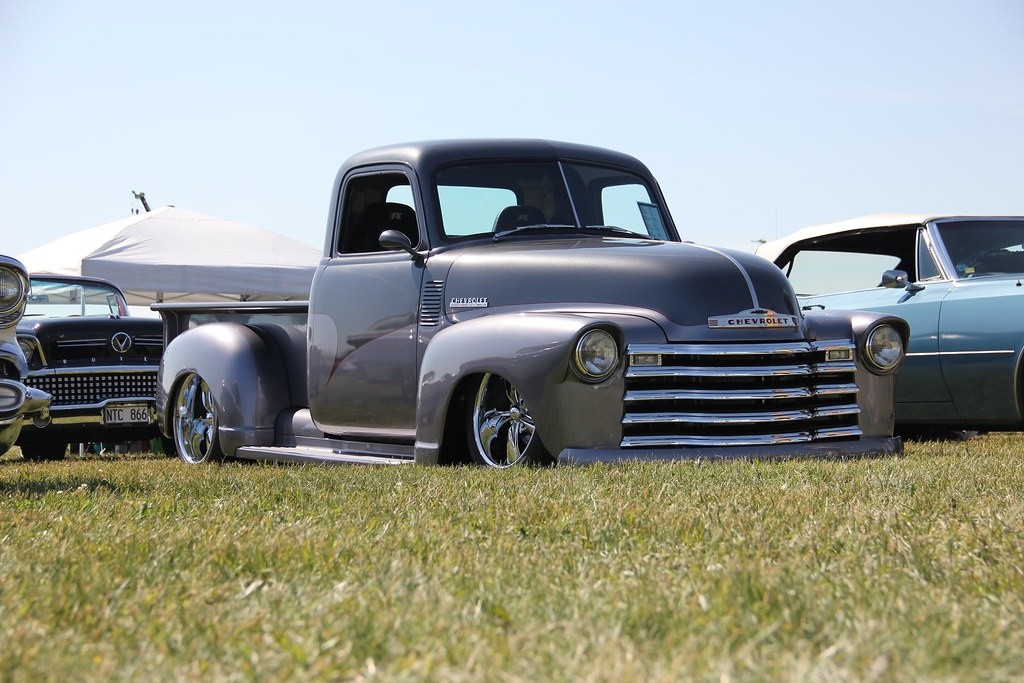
[755,213,1024,432]
[16,272,163,461]
[0,254,53,456]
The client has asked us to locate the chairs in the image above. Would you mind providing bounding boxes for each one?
[352,203,419,253]
[491,206,547,232]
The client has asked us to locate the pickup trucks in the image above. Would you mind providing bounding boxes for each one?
[150,137,912,467]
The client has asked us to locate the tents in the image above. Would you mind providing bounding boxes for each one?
[14,206,323,304]
